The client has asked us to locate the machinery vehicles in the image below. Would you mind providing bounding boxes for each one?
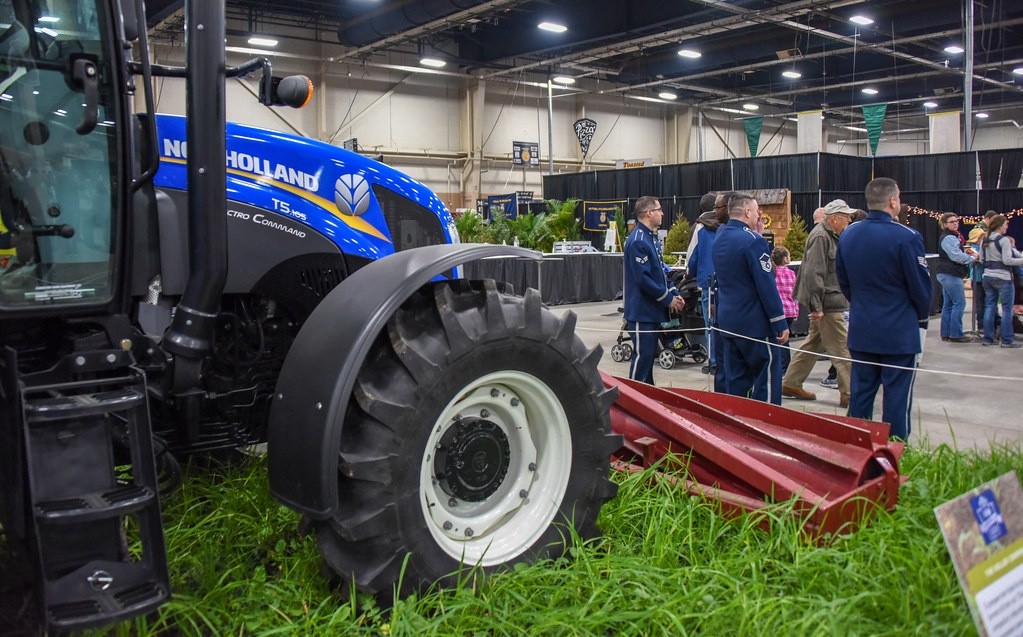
[0,0,911,637]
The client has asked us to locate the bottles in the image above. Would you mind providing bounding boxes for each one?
[502,240,507,246]
[514,236,519,247]
[562,239,566,253]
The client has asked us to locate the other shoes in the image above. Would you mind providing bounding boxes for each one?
[1000,339,1023,348]
[982,337,1000,346]
[942,334,971,342]
[702,365,717,375]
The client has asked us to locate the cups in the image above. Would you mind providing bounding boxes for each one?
[610,221,615,230]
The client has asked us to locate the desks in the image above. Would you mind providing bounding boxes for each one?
[462,251,624,306]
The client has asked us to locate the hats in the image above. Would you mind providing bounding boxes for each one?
[966,228,988,243]
[824,199,858,215]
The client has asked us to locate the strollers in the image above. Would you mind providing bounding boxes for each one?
[610,278,709,370]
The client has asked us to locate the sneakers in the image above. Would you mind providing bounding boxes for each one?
[840,398,849,407]
[782,385,816,399]
[820,376,839,389]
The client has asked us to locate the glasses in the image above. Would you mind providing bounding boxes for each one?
[643,206,662,214]
[713,204,728,212]
[946,220,959,225]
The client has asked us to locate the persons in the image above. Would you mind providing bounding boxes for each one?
[772,246,800,377]
[781,200,857,408]
[807,207,826,234]
[936,213,979,342]
[834,177,934,444]
[967,210,1023,347]
[624,196,686,385]
[848,209,868,225]
[683,192,791,406]
